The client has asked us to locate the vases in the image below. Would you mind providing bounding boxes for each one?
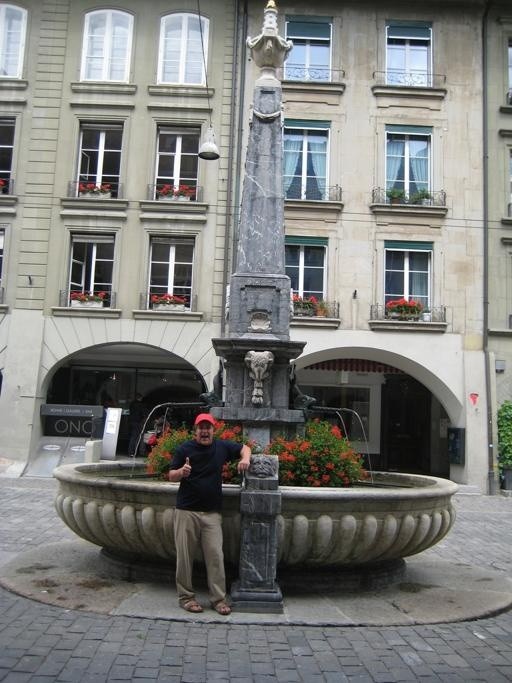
[67,299,185,312]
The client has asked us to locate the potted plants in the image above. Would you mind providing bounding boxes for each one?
[495,399,512,489]
[423,308,431,321]
[384,185,435,206]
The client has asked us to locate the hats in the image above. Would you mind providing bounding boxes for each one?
[195,413,215,426]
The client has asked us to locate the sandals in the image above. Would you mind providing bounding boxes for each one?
[179,599,203,612]
[211,601,230,615]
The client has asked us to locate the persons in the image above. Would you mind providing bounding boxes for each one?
[165,414,253,616]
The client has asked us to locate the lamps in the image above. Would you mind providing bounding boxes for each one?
[197,117,221,162]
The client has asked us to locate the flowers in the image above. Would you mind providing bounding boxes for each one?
[70,291,188,305]
[145,415,370,488]
[78,180,196,202]
[290,294,422,321]
